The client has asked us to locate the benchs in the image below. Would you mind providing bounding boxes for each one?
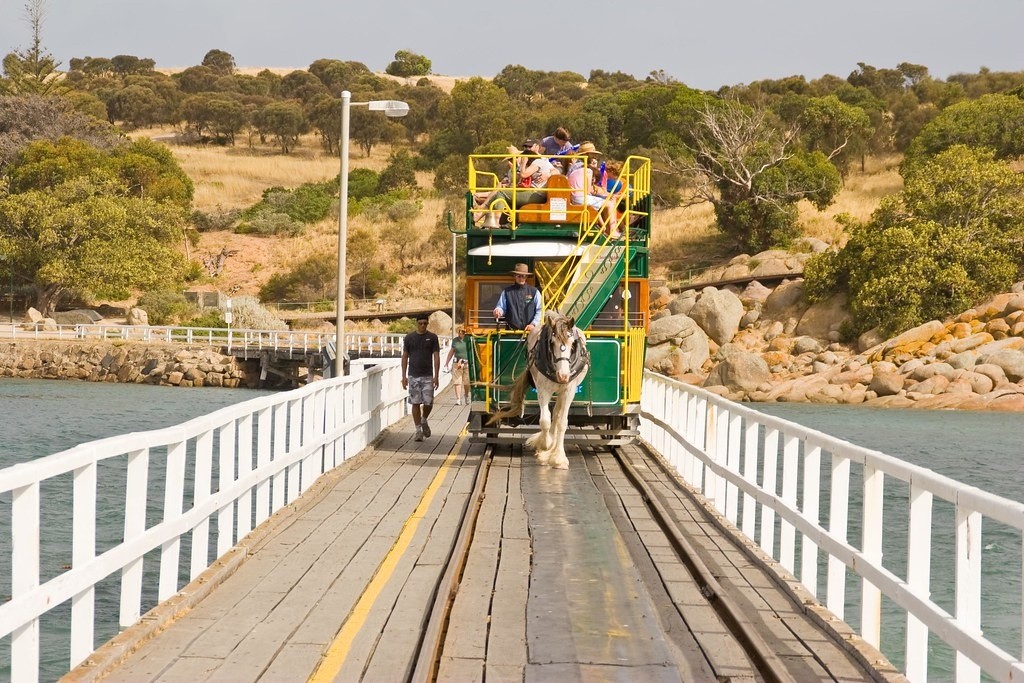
[516,175,600,226]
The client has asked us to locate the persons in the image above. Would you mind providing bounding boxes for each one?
[443,324,471,405]
[492,264,542,337]
[471,126,634,241]
[401,314,440,441]
[599,302,633,331]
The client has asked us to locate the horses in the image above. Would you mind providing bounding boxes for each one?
[485,312,591,471]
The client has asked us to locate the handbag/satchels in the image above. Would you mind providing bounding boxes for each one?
[591,185,608,196]
[516,175,532,188]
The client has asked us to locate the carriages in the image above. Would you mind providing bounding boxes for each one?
[449,155,652,471]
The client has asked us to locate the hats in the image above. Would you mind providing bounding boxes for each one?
[455,323,465,332]
[576,142,603,155]
[512,263,533,275]
[553,126,572,140]
[522,138,541,146]
[501,145,525,161]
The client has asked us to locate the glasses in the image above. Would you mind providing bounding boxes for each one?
[515,274,528,278]
[417,322,428,325]
[524,145,532,149]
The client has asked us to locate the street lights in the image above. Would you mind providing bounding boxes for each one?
[335,90,410,377]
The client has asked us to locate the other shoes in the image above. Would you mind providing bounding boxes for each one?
[420,417,431,438]
[464,393,470,405]
[415,431,423,441]
[454,401,461,405]
